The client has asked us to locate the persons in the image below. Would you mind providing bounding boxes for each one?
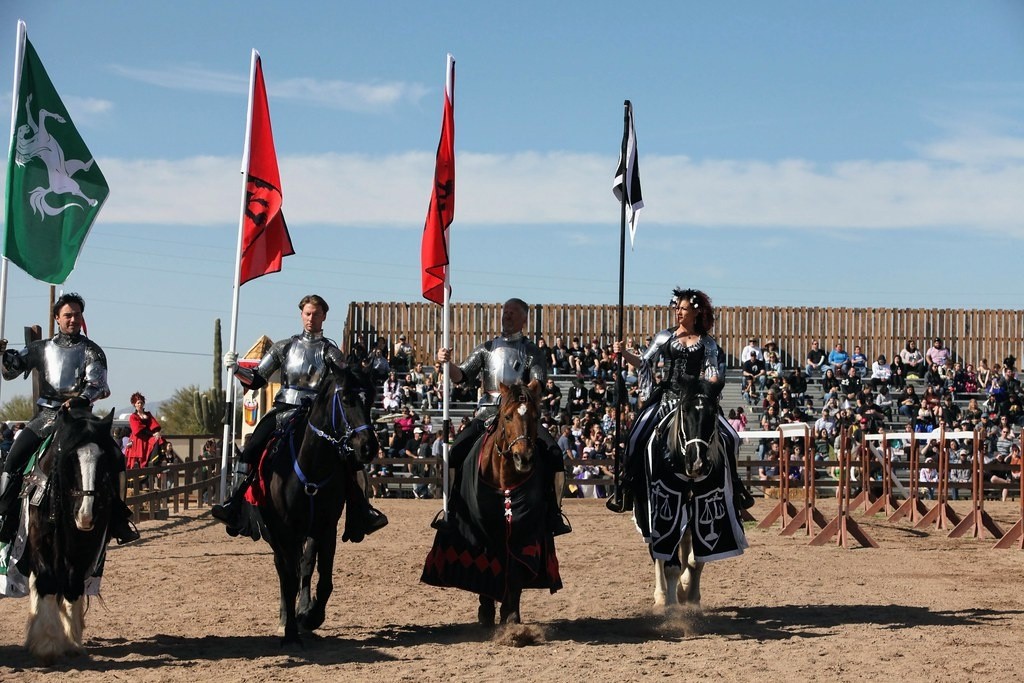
[1,421,26,467]
[612,287,748,512]
[339,329,1024,491]
[0,292,140,547]
[111,425,254,507]
[436,297,571,537]
[124,389,162,481]
[209,294,390,543]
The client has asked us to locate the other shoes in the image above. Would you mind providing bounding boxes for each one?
[554,516,570,535]
[742,489,755,509]
[368,510,387,532]
[119,525,139,542]
[212,502,237,527]
[609,499,633,511]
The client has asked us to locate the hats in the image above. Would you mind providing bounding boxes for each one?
[751,351,756,355]
[400,334,406,338]
[765,340,775,347]
[960,450,968,455]
[561,425,571,433]
[414,427,423,433]
[981,413,989,417]
[749,337,756,342]
[543,411,550,415]
[860,418,867,423]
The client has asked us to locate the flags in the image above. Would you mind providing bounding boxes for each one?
[420,52,456,311]
[0,17,110,288]
[237,47,296,290]
[612,99,645,251]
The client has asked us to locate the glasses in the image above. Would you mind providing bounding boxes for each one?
[795,449,801,450]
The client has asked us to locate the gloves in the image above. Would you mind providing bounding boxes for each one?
[224,351,239,374]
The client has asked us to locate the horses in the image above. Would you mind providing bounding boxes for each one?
[454,377,552,627]
[632,375,727,615]
[250,356,379,638]
[23,405,115,663]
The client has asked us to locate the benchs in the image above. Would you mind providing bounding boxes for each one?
[369,366,1024,502]
